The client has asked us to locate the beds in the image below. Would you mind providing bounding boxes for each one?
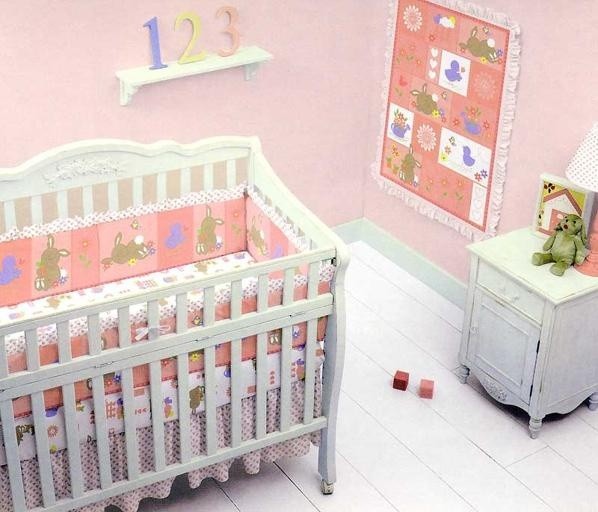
[0,137,348,512]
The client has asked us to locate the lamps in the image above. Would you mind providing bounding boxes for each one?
[566,122,598,237]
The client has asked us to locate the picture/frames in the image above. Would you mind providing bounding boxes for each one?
[533,172,595,246]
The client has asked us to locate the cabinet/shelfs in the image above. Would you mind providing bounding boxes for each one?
[458,227,598,438]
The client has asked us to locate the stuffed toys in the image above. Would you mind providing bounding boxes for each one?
[532,214,587,276]
